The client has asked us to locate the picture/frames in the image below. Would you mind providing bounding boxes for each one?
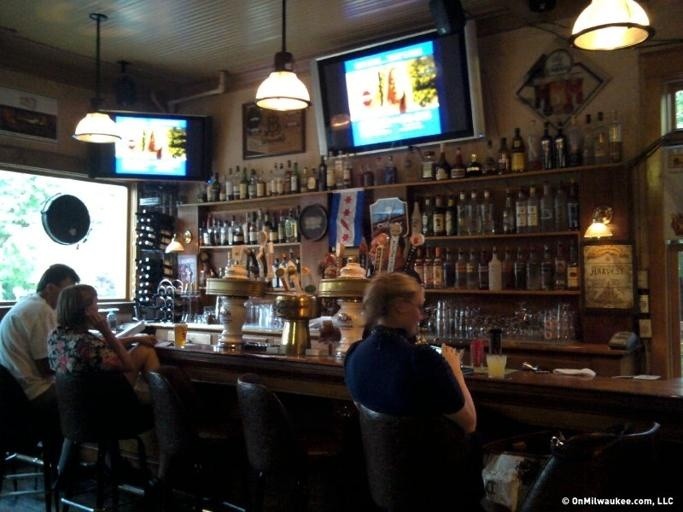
[242,101,306,160]
[512,37,613,132]
[580,239,637,315]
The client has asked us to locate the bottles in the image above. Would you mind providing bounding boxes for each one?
[414,248,424,284]
[582,115,594,164]
[257,178,265,198]
[318,155,327,190]
[489,248,502,291]
[270,211,278,241]
[554,123,568,167]
[540,182,554,230]
[336,152,345,188]
[424,249,433,287]
[136,209,175,227]
[458,192,472,236]
[608,110,622,162]
[257,211,264,243]
[284,210,298,240]
[229,215,235,244]
[309,169,318,189]
[594,113,608,163]
[526,249,539,289]
[135,258,162,306]
[147,227,174,238]
[436,152,449,180]
[226,169,234,200]
[273,258,278,287]
[301,168,307,190]
[240,168,248,199]
[199,220,221,246]
[376,159,385,184]
[554,242,567,291]
[221,222,230,245]
[145,242,166,250]
[515,247,526,290]
[567,117,579,164]
[554,182,566,228]
[218,268,223,279]
[483,190,493,234]
[328,153,336,188]
[294,206,301,241]
[470,190,481,234]
[137,229,146,248]
[515,187,527,233]
[470,341,484,368]
[479,252,489,288]
[484,141,495,173]
[344,154,353,187]
[107,309,116,333]
[433,196,445,235]
[243,213,256,244]
[208,173,219,201]
[234,167,242,199]
[468,155,483,177]
[420,152,436,180]
[288,252,298,275]
[387,157,397,184]
[543,301,577,341]
[433,247,443,287]
[278,216,284,242]
[234,224,244,245]
[568,240,580,289]
[292,162,300,192]
[541,123,556,167]
[445,249,454,288]
[503,247,514,289]
[278,258,288,288]
[567,178,579,231]
[363,169,373,186]
[455,251,467,286]
[541,246,556,289]
[285,161,292,194]
[526,121,538,170]
[147,235,172,245]
[224,254,237,275]
[423,197,433,236]
[504,188,514,233]
[527,185,538,232]
[498,138,511,174]
[263,215,269,242]
[436,300,483,338]
[248,171,257,199]
[445,194,456,234]
[411,203,422,236]
[200,269,207,288]
[511,127,526,171]
[467,252,479,287]
[265,162,283,196]
[451,148,466,178]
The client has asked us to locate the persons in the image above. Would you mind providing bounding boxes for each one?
[343,271,476,512]
[0,265,80,415]
[47,284,174,512]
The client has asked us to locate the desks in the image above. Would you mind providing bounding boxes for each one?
[158,340,683,447]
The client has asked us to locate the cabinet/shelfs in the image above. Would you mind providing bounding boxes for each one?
[135,160,643,376]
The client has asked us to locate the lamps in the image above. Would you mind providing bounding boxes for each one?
[254,52,312,112]
[165,230,192,254]
[584,205,614,239]
[72,97,122,144]
[568,0,657,53]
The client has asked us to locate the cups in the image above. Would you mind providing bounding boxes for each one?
[175,324,187,350]
[487,355,507,379]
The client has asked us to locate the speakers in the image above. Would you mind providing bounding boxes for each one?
[115,75,137,105]
[430,0,468,34]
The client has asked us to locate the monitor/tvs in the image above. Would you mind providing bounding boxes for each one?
[309,20,486,160]
[89,109,212,181]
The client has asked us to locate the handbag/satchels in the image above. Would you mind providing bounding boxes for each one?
[481,449,554,512]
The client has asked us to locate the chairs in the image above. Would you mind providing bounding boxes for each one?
[0,365,61,512]
[235,373,351,512]
[53,369,156,512]
[149,365,235,512]
[522,420,665,512]
[352,397,485,512]
